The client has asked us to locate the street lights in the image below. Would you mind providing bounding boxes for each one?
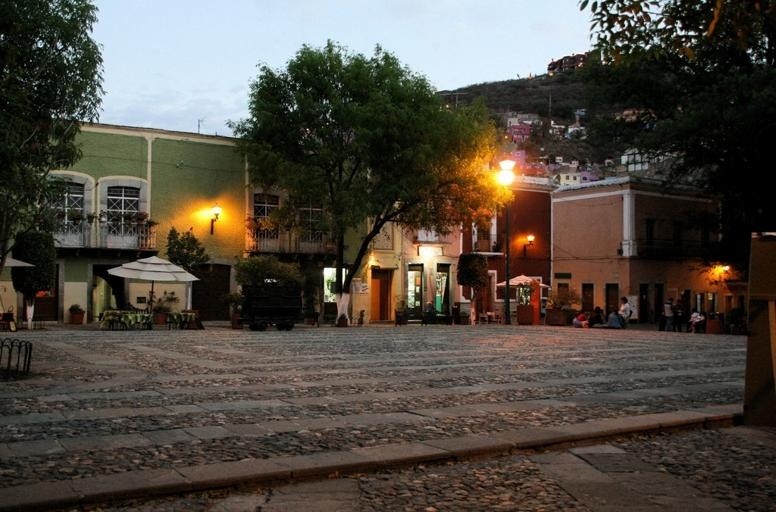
[491,151,516,324]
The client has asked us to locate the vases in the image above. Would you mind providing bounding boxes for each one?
[154,312,167,324]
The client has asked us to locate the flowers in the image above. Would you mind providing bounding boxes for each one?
[146,289,179,311]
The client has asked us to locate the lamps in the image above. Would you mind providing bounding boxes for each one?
[523,234,536,257]
[210,202,223,235]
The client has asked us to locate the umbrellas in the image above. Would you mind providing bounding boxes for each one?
[105,255,202,315]
[0,252,38,272]
[493,273,550,295]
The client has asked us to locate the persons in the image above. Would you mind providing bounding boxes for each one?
[126,301,135,310]
[559,299,569,310]
[161,290,168,301]
[542,298,554,325]
[473,239,480,251]
[491,239,497,251]
[419,300,434,326]
[166,290,176,307]
[395,299,406,325]
[570,292,708,337]
[156,297,161,305]
[310,286,321,328]
[147,290,157,310]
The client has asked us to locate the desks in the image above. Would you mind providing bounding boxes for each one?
[101,307,154,330]
[166,311,196,329]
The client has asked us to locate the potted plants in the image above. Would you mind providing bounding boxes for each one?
[515,283,581,326]
[69,303,86,324]
[222,292,245,330]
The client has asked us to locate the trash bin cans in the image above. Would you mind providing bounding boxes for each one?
[706,312,724,334]
